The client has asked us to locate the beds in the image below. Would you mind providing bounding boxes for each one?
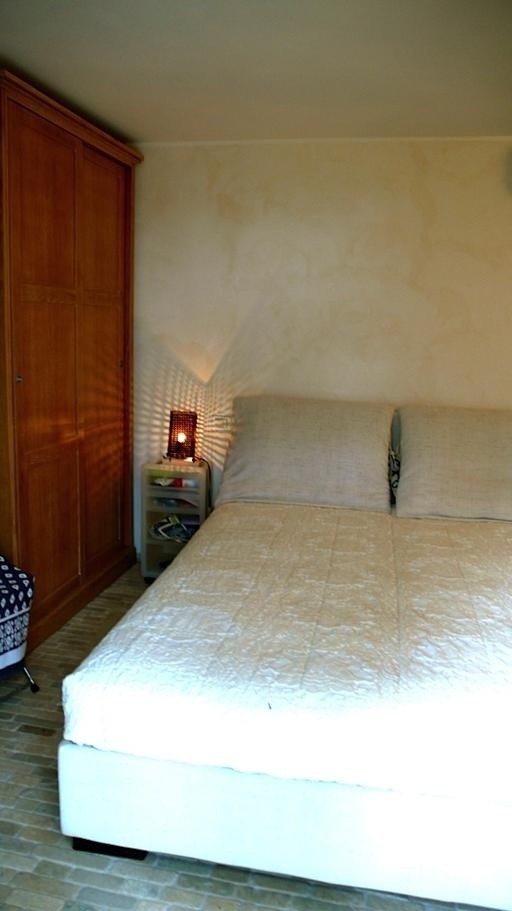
[52,497,511,903]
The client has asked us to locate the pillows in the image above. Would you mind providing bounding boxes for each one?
[395,404,512,520]
[214,396,395,514]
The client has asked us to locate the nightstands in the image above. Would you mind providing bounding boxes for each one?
[139,460,208,583]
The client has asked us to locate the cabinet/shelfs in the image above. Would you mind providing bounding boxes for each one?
[0,67,143,660]
